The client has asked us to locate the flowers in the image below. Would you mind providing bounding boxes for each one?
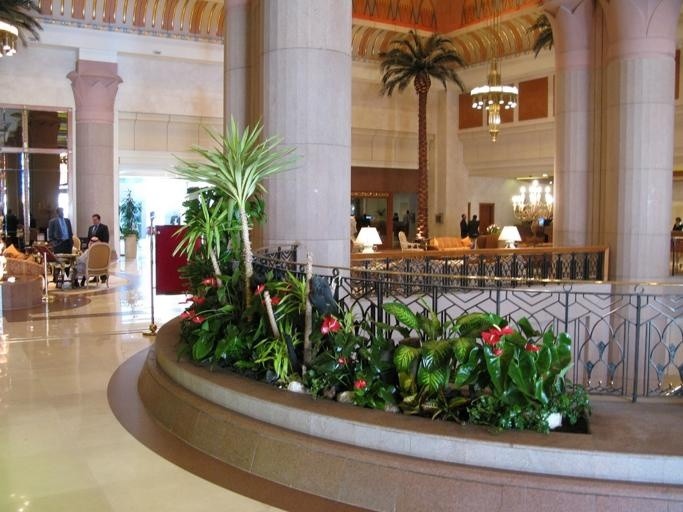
[485,223,501,233]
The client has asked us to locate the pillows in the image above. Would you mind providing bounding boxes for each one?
[430,237,439,248]
[461,236,473,248]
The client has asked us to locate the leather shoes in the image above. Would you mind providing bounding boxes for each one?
[89,275,106,283]
[72,281,85,287]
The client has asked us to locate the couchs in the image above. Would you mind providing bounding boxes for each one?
[426,236,474,252]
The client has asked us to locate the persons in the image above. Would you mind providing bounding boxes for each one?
[459,215,467,239]
[2,209,37,253]
[70,235,101,287]
[355,209,410,240]
[468,215,480,238]
[84,214,109,283]
[47,208,79,283]
[673,217,683,231]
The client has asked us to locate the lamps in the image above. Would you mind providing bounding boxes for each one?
[354,226,383,254]
[470,1,521,143]
[498,225,522,249]
[510,179,557,242]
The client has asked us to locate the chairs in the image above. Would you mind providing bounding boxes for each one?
[75,242,111,289]
[399,231,424,252]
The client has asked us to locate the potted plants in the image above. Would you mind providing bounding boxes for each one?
[117,191,141,259]
